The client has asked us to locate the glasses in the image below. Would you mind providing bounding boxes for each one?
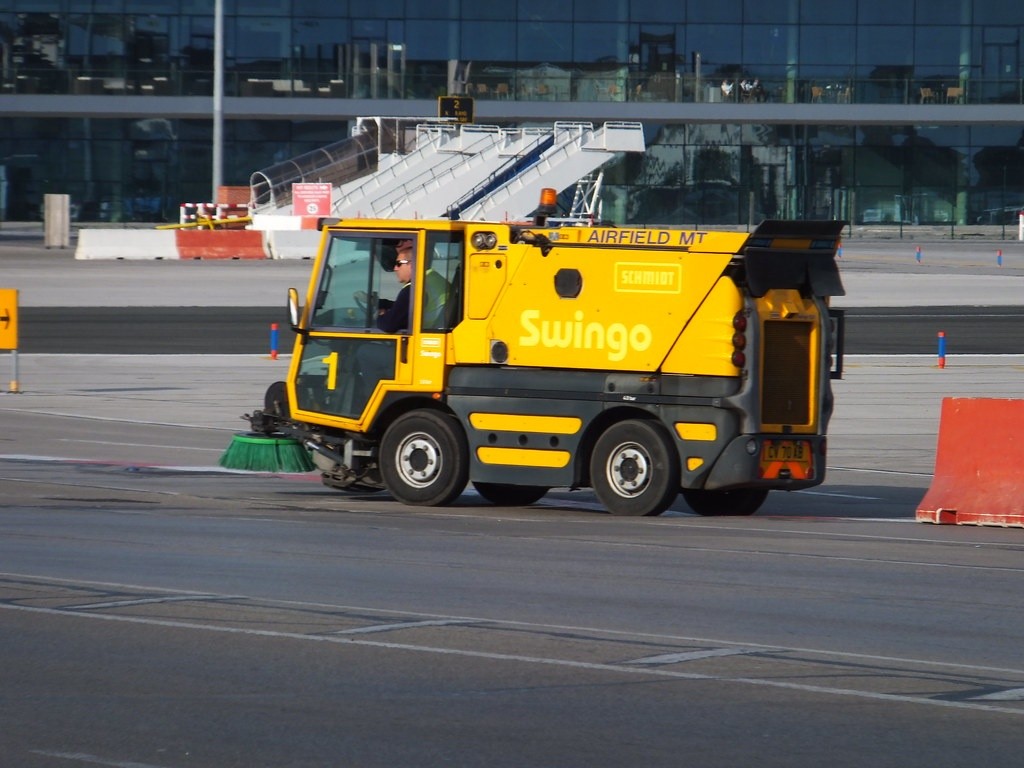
[395,260,410,267]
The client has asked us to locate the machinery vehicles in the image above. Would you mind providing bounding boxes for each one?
[216,187,846,519]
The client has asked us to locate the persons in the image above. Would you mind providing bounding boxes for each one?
[310,239,457,421]
